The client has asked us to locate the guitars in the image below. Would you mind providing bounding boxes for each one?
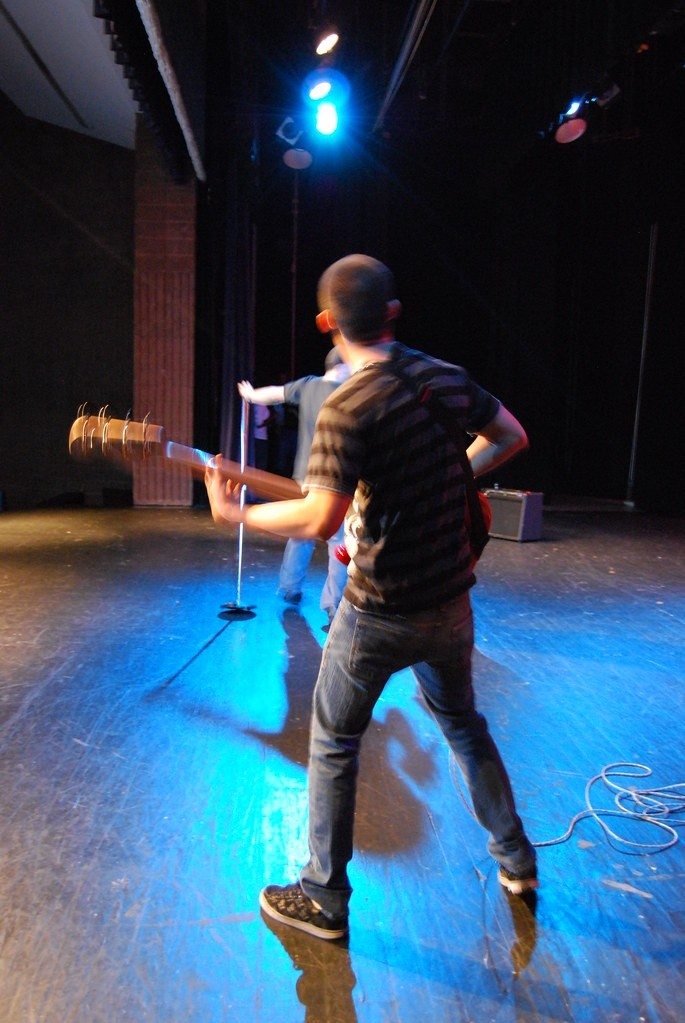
[67,401,494,572]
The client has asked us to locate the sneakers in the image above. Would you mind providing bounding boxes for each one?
[260,880,348,939]
[496,861,539,896]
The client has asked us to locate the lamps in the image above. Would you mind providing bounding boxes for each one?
[276,116,305,146]
[548,113,587,144]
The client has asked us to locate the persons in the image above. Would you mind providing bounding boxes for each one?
[237,345,351,627]
[204,249,539,942]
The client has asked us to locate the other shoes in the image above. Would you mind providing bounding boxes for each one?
[281,589,300,608]
